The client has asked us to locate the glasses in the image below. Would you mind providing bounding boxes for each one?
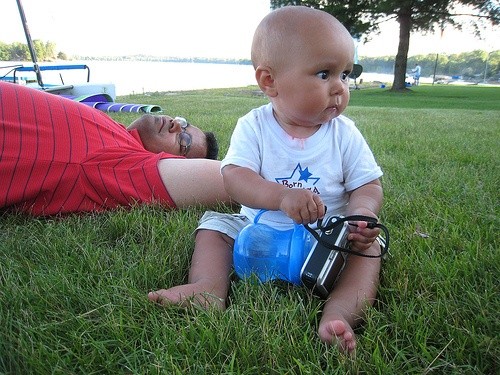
[175,116,192,156]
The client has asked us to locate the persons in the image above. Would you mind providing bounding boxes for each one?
[0,81,231,216]
[147,6,387,361]
[412,63,420,86]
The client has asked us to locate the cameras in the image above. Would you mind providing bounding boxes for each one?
[300,215,352,299]
